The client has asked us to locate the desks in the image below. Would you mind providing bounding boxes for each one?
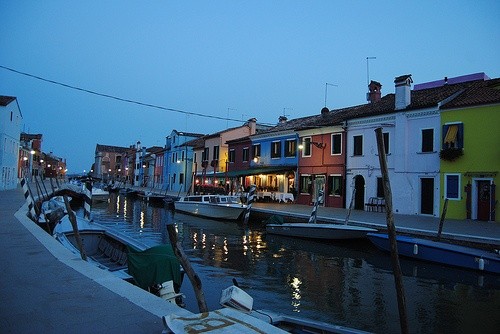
[258,191,294,203]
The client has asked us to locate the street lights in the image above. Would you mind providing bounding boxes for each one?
[179,130,187,193]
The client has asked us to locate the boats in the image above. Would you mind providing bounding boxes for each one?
[218,279,388,334]
[265,217,379,241]
[94,182,135,195]
[138,194,166,202]
[51,196,73,205]
[39,200,75,224]
[90,187,109,205]
[173,194,253,223]
[163,196,182,209]
[52,214,188,286]
[366,232,500,275]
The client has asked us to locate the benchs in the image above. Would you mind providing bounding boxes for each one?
[365,197,386,212]
